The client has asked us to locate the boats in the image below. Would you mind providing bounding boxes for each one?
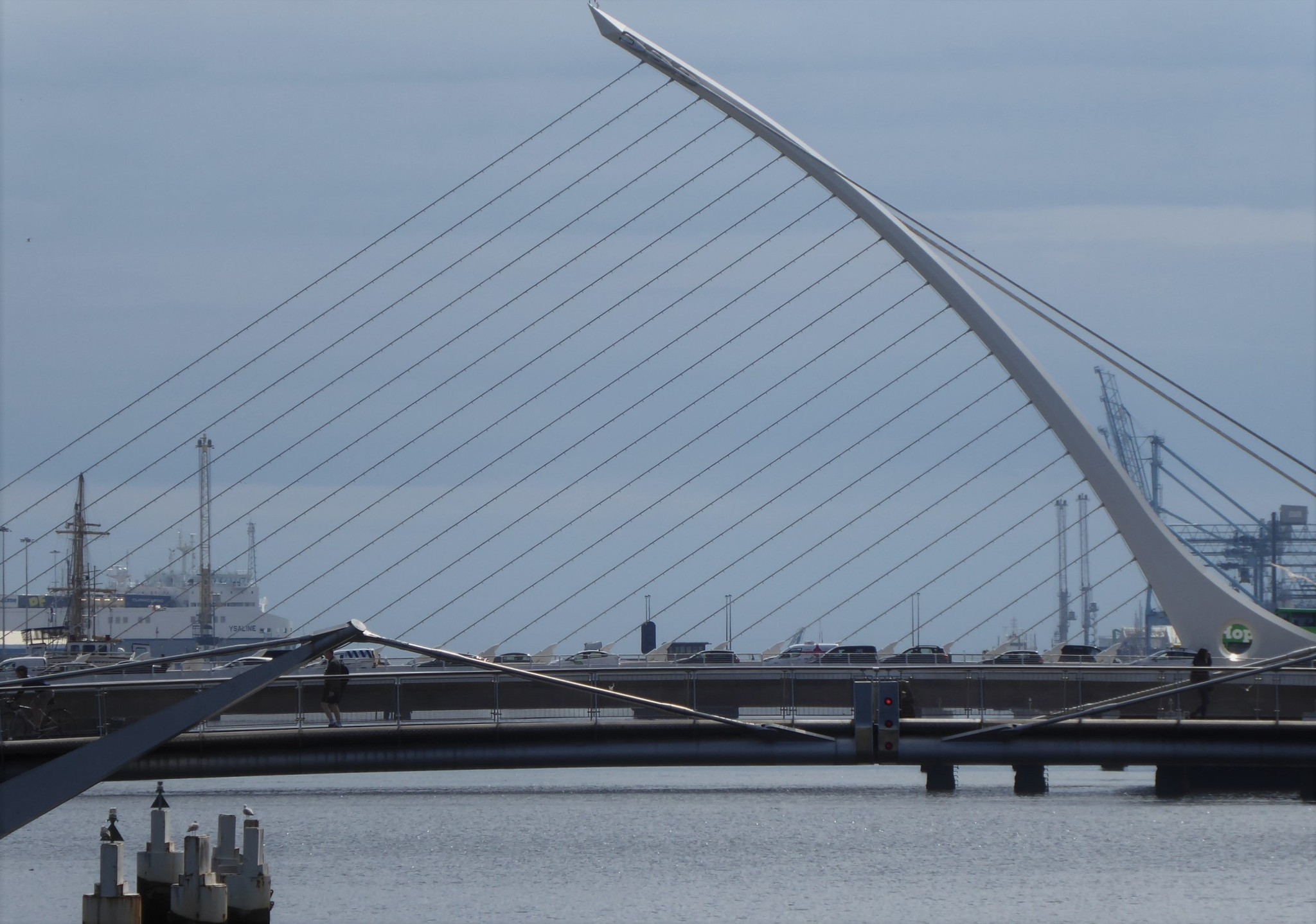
[0,527,383,669]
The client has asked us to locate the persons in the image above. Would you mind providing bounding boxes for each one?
[159,654,169,668]
[899,680,916,719]
[14,665,54,739]
[1186,648,1213,719]
[321,649,342,727]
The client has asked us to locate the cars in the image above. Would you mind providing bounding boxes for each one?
[1129,643,1198,667]
[97,660,168,672]
[210,656,274,666]
[410,652,489,667]
[546,650,620,666]
[37,662,98,677]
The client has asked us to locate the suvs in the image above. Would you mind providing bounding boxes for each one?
[975,651,1043,664]
[670,649,740,664]
[484,652,534,663]
[878,645,952,664]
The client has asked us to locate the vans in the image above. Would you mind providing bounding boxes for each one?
[304,648,377,668]
[0,657,47,671]
[1056,644,1121,663]
[762,640,840,664]
[809,645,878,664]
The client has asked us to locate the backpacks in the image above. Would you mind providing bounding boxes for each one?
[331,659,349,692]
[1190,664,1202,683]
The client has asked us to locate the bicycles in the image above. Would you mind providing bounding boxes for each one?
[0,694,76,741]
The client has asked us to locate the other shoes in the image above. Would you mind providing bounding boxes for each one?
[334,721,342,728]
[328,721,336,728]
